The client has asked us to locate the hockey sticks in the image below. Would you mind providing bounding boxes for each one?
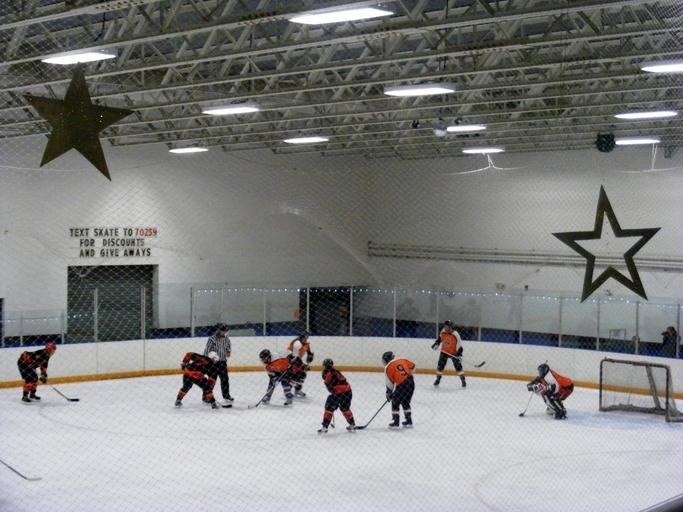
[437,348,486,367]
[248,358,295,408]
[179,363,232,408]
[519,360,548,416]
[0,460,44,480]
[353,400,388,429]
[45,381,80,401]
[330,412,335,428]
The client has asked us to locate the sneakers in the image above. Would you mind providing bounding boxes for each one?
[390,418,412,427]
[175,398,183,406]
[21,393,40,403]
[433,379,441,385]
[317,427,328,433]
[462,379,466,386]
[262,394,271,402]
[347,423,355,431]
[284,390,305,405]
[210,397,233,408]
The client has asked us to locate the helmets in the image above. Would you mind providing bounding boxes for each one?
[300,331,309,339]
[260,350,270,358]
[208,350,220,362]
[381,352,394,363]
[45,343,56,353]
[538,365,549,377]
[323,359,334,368]
[444,320,456,330]
[219,323,230,333]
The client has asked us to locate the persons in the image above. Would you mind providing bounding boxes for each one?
[202,323,234,403]
[18,342,58,403]
[260,348,301,406]
[382,351,415,427]
[316,358,355,432]
[527,363,574,419]
[174,351,223,407]
[432,320,467,387]
[662,327,680,356]
[288,329,314,397]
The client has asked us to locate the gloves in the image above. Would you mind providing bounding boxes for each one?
[306,353,314,363]
[271,376,280,386]
[433,340,439,350]
[385,388,393,402]
[458,351,463,357]
[39,375,48,384]
